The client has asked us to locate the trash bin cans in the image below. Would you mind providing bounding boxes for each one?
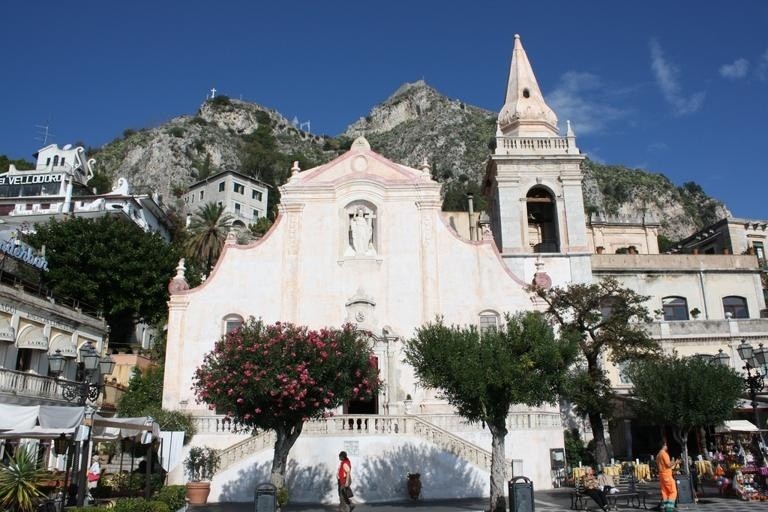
[254,482,277,512]
[508,477,535,512]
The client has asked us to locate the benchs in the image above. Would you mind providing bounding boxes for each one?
[568,471,648,511]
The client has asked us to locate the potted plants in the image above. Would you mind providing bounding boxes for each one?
[183,444,221,506]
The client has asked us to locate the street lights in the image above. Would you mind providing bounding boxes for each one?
[709,338,767,442]
[47,338,117,509]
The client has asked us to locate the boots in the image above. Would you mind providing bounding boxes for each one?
[659,500,676,512]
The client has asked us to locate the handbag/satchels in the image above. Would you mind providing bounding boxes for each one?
[341,487,355,500]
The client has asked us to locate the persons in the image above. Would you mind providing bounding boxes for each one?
[675,455,683,469]
[582,467,610,512]
[353,208,372,256]
[133,459,154,475]
[628,245,637,255]
[656,436,681,512]
[746,247,755,255]
[596,247,603,255]
[336,451,355,512]
[700,438,767,501]
[721,247,730,254]
